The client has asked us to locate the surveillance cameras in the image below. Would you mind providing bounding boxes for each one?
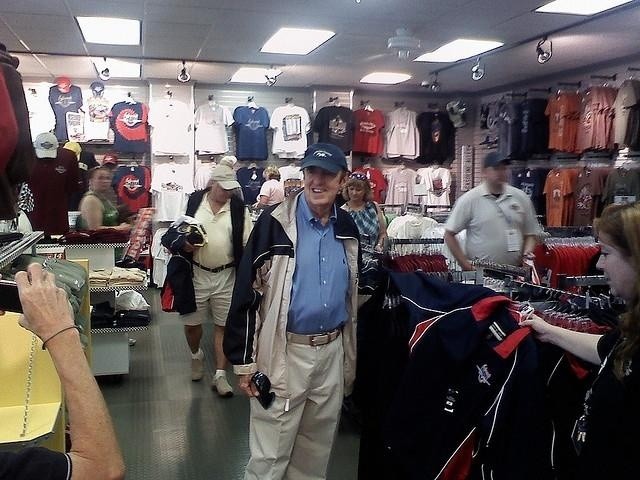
[421,81,430,88]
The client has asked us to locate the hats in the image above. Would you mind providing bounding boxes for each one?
[484,152,510,167]
[35,133,59,159]
[102,156,117,166]
[212,166,241,191]
[300,143,346,173]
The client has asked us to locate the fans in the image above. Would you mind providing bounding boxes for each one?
[332,20,437,71]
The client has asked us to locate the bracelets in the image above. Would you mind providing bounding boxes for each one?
[41,325,84,351]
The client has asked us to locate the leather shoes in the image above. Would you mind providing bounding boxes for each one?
[191,352,205,381]
[212,376,232,397]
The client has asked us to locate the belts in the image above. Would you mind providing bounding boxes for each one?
[287,330,340,346]
[192,260,235,272]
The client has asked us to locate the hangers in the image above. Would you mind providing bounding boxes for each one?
[362,231,639,333]
[248,161,261,170]
[123,90,261,110]
[333,96,375,112]
[362,160,406,171]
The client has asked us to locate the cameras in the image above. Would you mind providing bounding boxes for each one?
[0,279,24,314]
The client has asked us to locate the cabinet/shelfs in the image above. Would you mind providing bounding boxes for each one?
[19,241,153,383]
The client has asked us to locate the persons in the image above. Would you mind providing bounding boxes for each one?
[226,141,366,479]
[163,165,252,400]
[80,167,133,234]
[343,171,389,270]
[512,202,639,477]
[0,253,136,480]
[257,164,287,207]
[441,152,550,281]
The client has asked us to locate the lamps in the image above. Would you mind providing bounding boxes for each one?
[4,48,288,89]
[426,1,639,95]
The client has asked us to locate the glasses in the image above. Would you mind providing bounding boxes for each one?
[349,173,367,180]
